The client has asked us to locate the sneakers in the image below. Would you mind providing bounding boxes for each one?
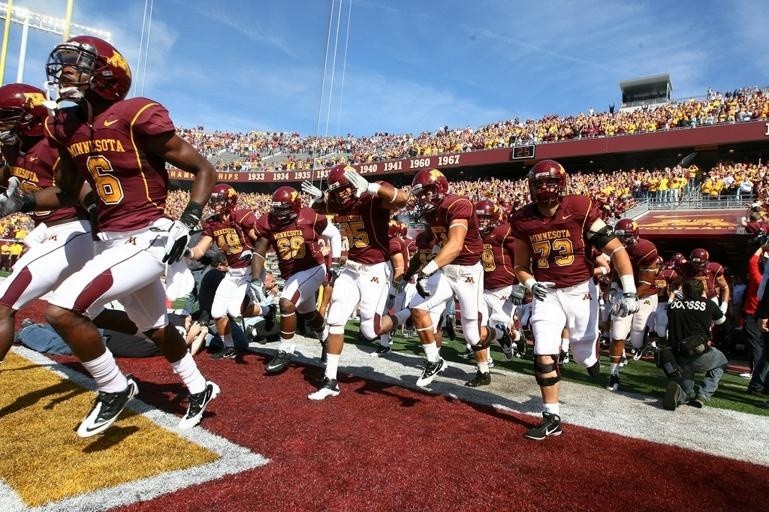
[247,325,255,341]
[465,370,490,387]
[619,356,628,367]
[265,350,293,372]
[524,411,562,440]
[404,303,415,330]
[264,305,276,331]
[307,377,340,400]
[633,348,642,359]
[445,315,456,341]
[368,337,393,356]
[458,323,527,367]
[691,396,705,407]
[664,382,681,410]
[558,351,569,365]
[208,347,237,360]
[416,357,448,386]
[180,382,220,427]
[321,339,328,362]
[607,374,619,391]
[74,375,139,438]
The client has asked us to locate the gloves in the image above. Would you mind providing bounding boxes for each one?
[510,286,526,305]
[527,279,555,302]
[239,250,253,261]
[416,259,438,299]
[395,274,408,294]
[301,180,325,209]
[345,169,378,199]
[1,177,27,218]
[610,274,640,317]
[248,279,267,303]
[163,215,203,264]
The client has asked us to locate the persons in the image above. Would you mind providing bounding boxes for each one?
[664,277,728,410]
[507,160,639,441]
[221,85,769,398]
[187,165,412,401]
[1,36,221,437]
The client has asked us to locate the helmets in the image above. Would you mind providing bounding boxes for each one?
[411,168,448,214]
[327,164,357,209]
[46,37,131,101]
[270,186,301,226]
[615,219,639,247]
[528,161,566,208]
[0,83,48,155]
[208,184,238,217]
[657,249,709,271]
[475,201,504,232]
